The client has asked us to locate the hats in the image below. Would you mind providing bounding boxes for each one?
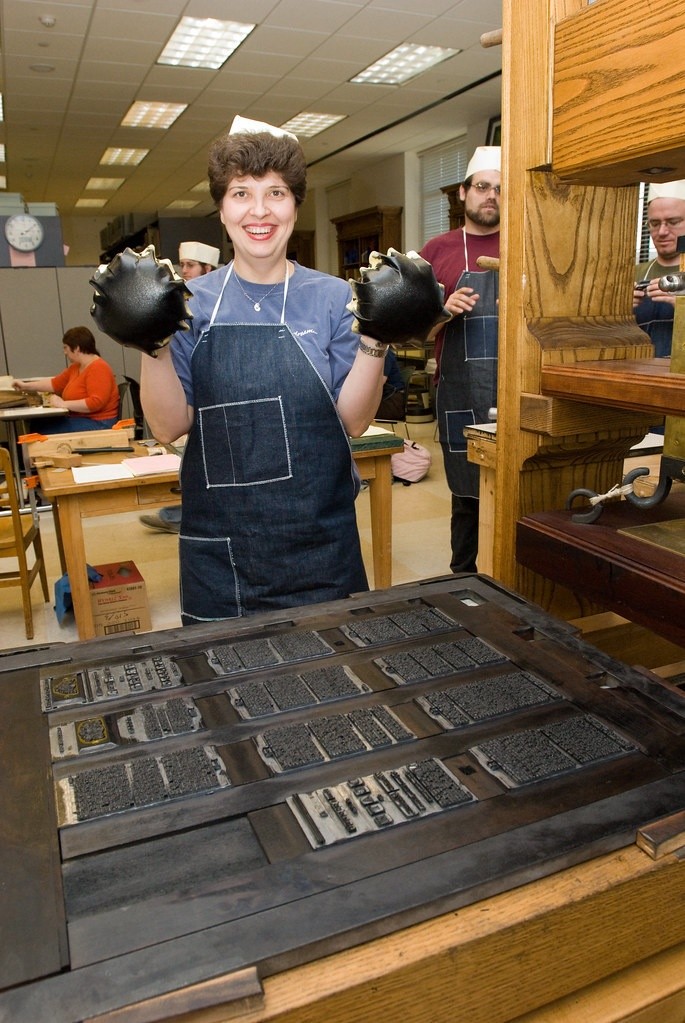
[647,178,685,203]
[464,146,501,179]
[179,241,220,266]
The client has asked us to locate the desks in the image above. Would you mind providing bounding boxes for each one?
[0,376,75,516]
[36,426,407,638]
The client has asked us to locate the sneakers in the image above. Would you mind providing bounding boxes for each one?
[139,515,181,533]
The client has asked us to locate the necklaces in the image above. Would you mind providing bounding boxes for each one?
[233,269,282,312]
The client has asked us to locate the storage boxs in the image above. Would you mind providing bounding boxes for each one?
[24,424,130,461]
[91,559,154,639]
[26,201,60,217]
[0,192,25,202]
[0,202,27,215]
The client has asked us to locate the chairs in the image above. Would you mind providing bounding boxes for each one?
[118,382,130,419]
[0,446,51,640]
[121,374,143,438]
[371,363,416,440]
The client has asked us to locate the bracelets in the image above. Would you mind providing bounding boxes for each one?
[358,341,387,358]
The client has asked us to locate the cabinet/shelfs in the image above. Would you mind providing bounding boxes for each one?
[328,205,404,282]
[457,0,685,690]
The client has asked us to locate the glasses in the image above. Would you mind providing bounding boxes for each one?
[644,218,685,229]
[464,182,500,194]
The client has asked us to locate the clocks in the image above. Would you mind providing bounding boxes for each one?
[4,214,46,252]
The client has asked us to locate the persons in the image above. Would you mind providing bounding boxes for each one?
[12,326,120,433]
[90,114,453,627]
[634,183,685,355]
[138,242,221,534]
[422,146,502,576]
[383,350,400,391]
